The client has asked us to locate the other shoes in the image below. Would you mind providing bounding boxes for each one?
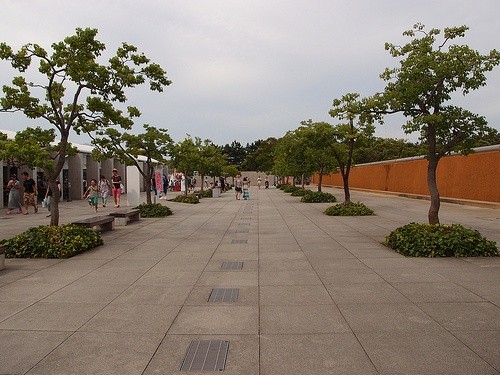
[115,204,117,207]
[35,206,38,212]
[7,212,11,214]
[117,205,120,208]
[24,212,28,215]
[19,208,22,213]
[103,204,105,207]
[46,214,51,217]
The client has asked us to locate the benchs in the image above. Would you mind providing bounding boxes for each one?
[71,215,115,231]
[109,208,141,226]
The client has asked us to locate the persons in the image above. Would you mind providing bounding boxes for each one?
[190,176,196,191]
[169,179,179,191]
[187,178,190,193]
[205,177,209,188]
[159,191,163,198]
[264,176,269,189]
[98,175,111,207]
[82,178,99,213]
[111,168,122,208]
[163,176,169,195]
[44,177,61,217]
[234,174,250,200]
[215,180,219,186]
[152,177,157,195]
[219,176,225,192]
[21,171,38,215]
[257,175,262,189]
[6,173,22,215]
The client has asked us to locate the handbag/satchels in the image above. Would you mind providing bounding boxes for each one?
[235,187,241,191]
[120,183,125,194]
[41,198,48,208]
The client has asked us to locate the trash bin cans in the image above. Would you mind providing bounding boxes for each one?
[212,188,221,198]
